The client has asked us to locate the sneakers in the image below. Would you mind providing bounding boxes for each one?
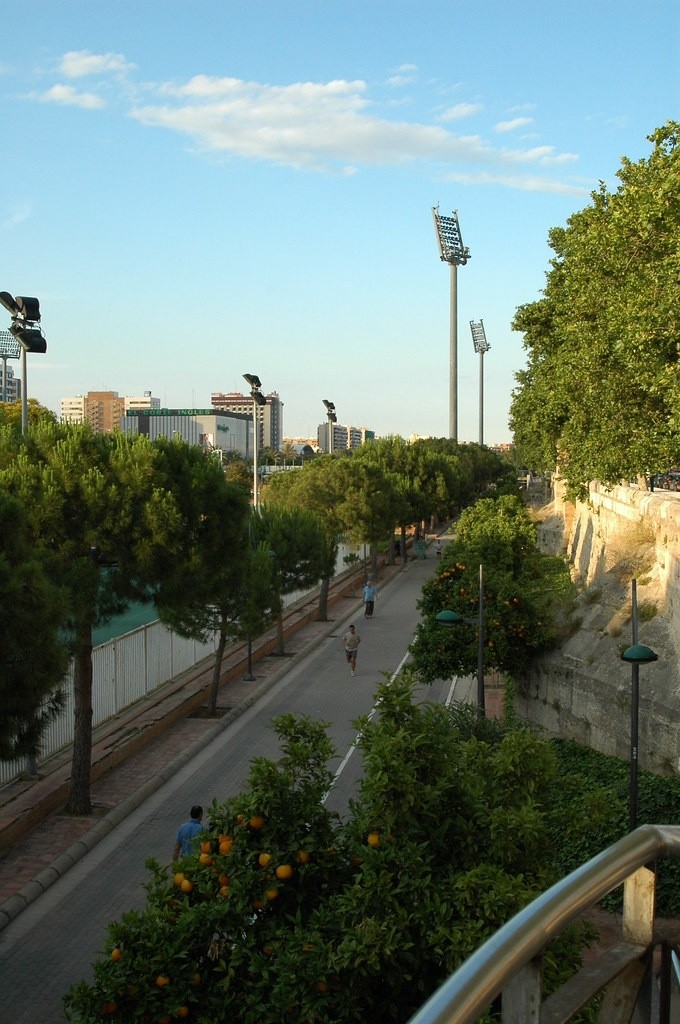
[351,671,354,677]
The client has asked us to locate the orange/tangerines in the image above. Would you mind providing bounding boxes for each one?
[100,814,380,1024]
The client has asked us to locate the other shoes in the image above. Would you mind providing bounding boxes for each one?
[365,615,372,619]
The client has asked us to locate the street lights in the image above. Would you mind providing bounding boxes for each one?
[434,563,486,722]
[242,373,267,509]
[323,399,337,457]
[619,578,659,831]
[0,291,48,435]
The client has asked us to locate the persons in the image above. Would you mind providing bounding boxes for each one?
[435,540,443,560]
[173,805,207,903]
[343,625,361,677]
[393,540,401,556]
[363,581,378,619]
